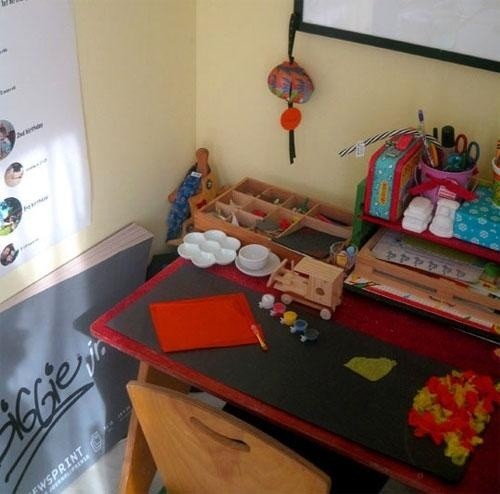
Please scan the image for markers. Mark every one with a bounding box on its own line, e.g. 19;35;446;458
441;125;455;166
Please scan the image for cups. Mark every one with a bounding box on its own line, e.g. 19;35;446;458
492;157;500;209
330;242;358;270
238;243;270;270
420;154;476;208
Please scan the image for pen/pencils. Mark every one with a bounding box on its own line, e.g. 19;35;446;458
238;295;267;350
417;111;438;170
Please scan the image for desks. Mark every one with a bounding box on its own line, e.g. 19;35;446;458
87;256;500;494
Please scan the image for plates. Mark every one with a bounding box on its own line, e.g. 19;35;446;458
235;252;281;278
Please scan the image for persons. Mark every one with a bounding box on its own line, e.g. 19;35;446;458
0;126;12;155
1;247;15;265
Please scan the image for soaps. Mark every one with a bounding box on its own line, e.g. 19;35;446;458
433;216;451;232
409;196;431;213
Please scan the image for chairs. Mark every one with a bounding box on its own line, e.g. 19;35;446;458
123;378;332;494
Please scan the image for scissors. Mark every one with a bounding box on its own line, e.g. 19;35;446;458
455;134;480;170
